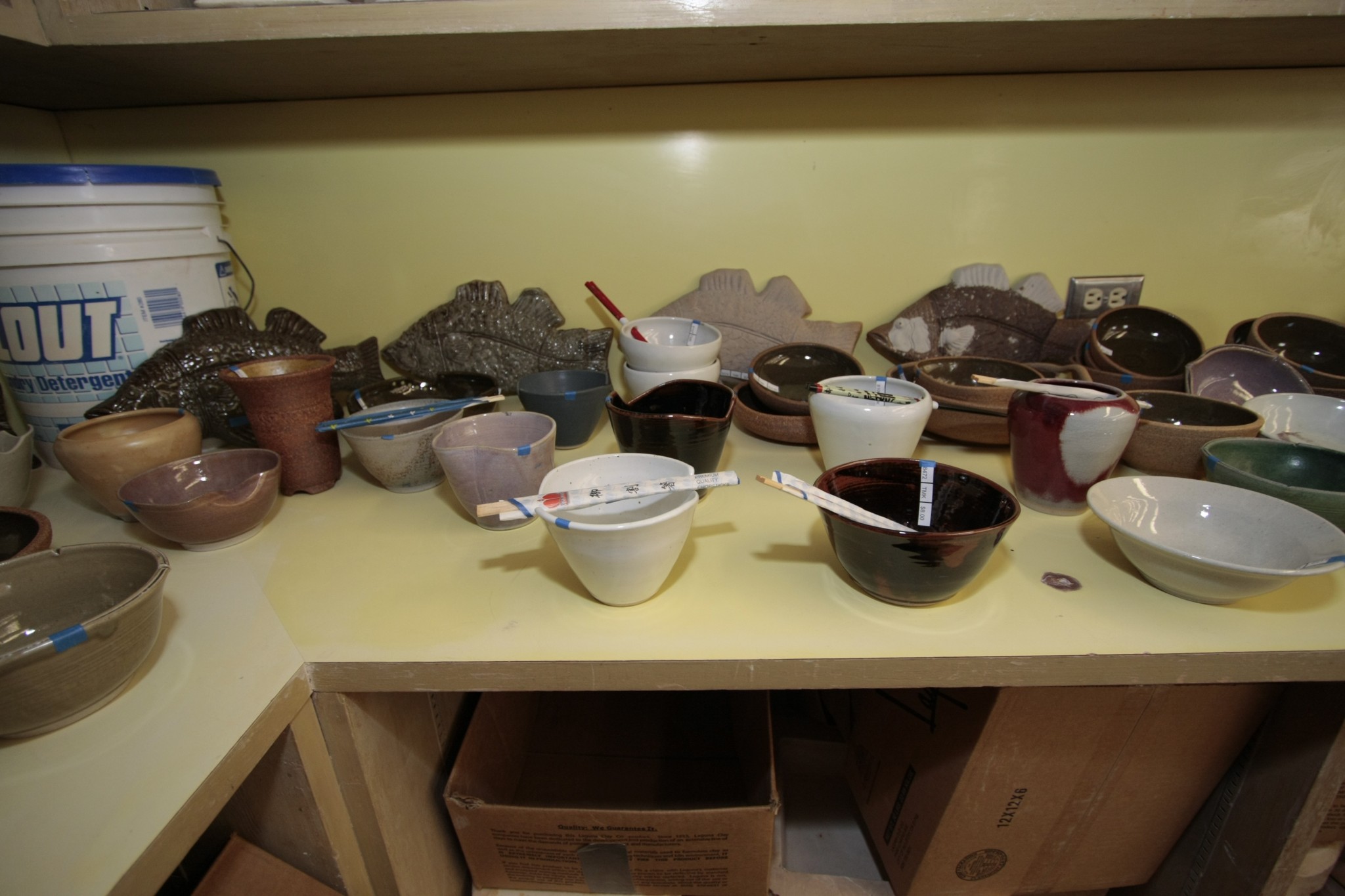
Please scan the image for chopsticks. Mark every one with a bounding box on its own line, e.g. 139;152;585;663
756;475;914;532
317;393;506;431
804;381;1008;418
585;281;649;343
477;471;739;518
970;372;1154;411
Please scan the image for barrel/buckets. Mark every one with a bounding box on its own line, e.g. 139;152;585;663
0;164;257;471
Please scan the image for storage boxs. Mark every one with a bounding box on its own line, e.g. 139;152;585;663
440;693;784;896
168;831;344;896
1285;778;1345;896
842;681;1278;896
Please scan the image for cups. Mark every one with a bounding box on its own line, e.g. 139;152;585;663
538;452;699;608
807;374;932;470
431;410;557;532
1008;377;1141;516
219;353;344;498
603;379;736;500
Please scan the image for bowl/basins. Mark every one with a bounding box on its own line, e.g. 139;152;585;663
0;408;280;740
338;316;736;606
732;305;1345;609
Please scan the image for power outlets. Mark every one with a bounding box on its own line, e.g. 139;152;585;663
1063;274;1146;318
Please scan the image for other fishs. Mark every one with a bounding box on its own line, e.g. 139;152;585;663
380;279;613;396
85;305;386;434
867;262;1091;366
648;268;863;382
